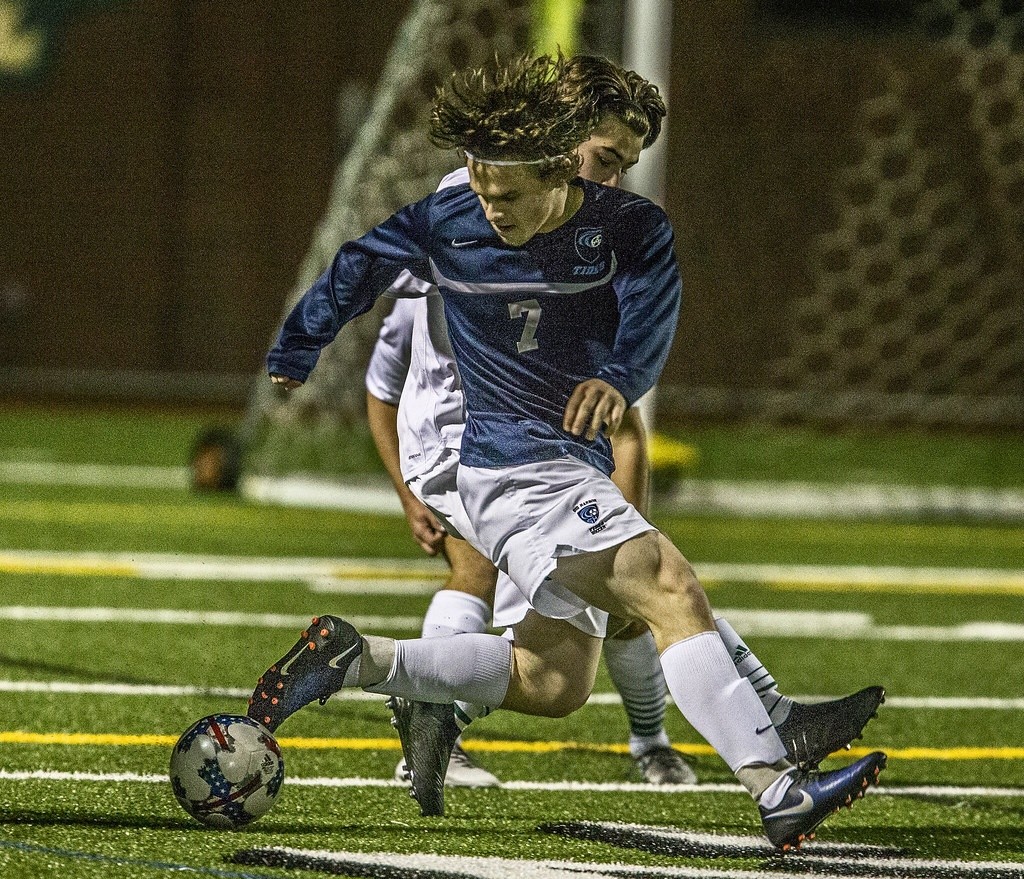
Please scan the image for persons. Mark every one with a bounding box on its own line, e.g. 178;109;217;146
268;43;888;851
385;53;885;817
366;298;697;788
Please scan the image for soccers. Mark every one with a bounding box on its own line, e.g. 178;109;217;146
169;712;285;829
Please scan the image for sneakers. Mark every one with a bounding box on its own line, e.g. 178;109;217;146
757;752;888;853
395;741;499;788
246;614;364;733
778;685;885;769
385;694;461;817
631;738;699;784
